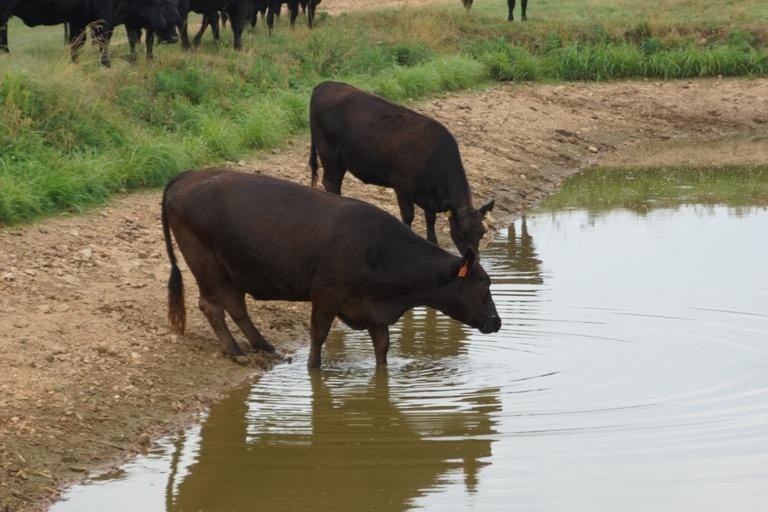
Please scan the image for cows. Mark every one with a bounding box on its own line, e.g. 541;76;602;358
309;81;495;261
462;0;531;22
0;0;322;65
161;166;502;370
